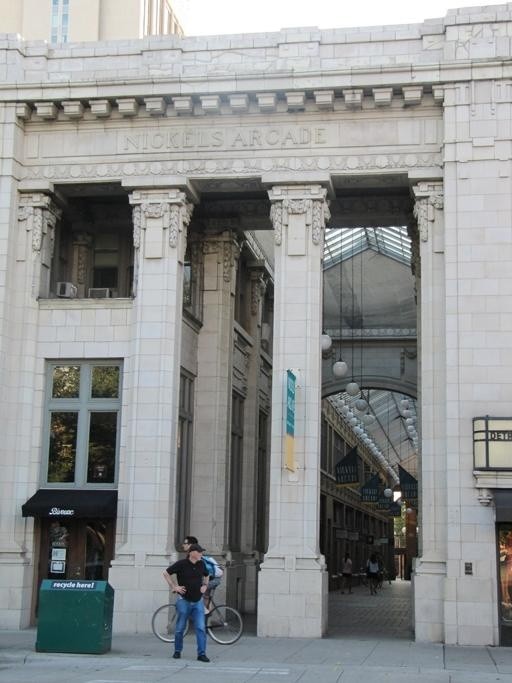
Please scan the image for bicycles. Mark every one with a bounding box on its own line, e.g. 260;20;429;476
152;586;244;645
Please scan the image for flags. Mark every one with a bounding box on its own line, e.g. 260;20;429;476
376;486;402;518
334;447;360;490
398;461;419;511
360;473;380;504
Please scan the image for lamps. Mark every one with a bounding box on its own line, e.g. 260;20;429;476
322;228;419;514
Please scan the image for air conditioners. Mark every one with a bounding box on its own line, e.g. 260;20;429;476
88;288;112;298
56;282;77;298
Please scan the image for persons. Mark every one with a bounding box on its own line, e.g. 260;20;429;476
367;551;385;595
341;552;354;594
182;536;223;615
162;544;209;662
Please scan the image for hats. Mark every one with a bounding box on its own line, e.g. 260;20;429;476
189;545;205;552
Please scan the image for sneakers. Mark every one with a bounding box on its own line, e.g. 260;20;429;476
173;652;180;658
198;655;209;662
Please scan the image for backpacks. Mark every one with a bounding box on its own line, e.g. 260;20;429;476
201;556;223;577
